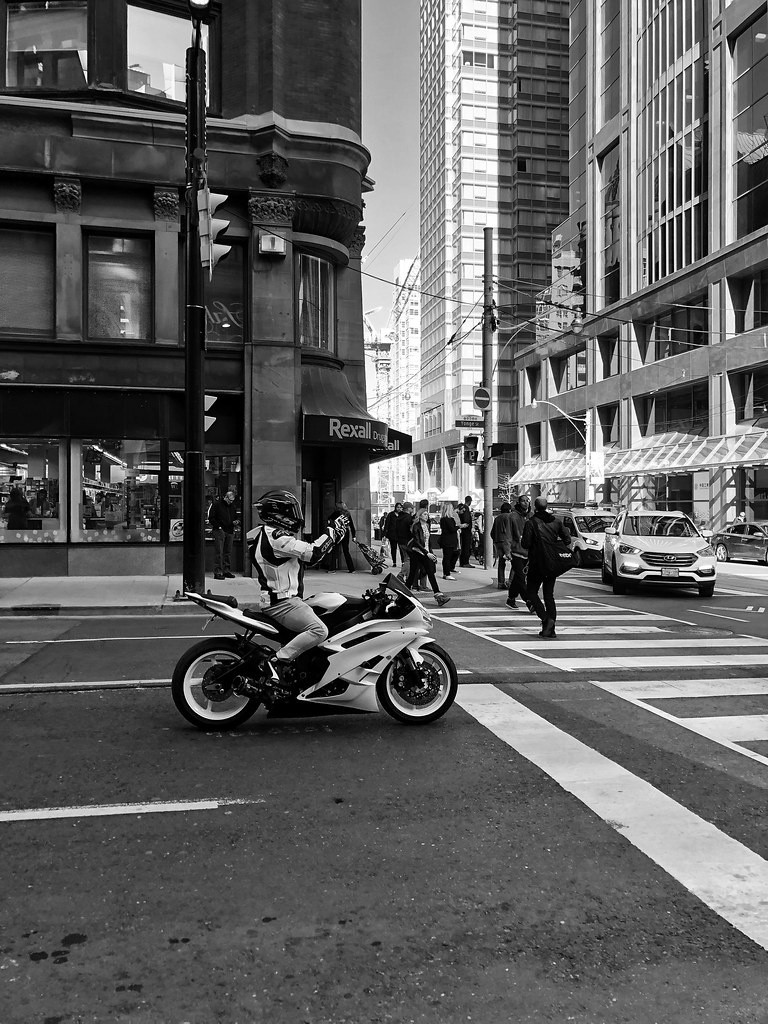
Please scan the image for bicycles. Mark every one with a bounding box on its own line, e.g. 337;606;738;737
473;540;496;568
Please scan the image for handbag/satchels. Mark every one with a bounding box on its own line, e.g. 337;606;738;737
541;540;575;576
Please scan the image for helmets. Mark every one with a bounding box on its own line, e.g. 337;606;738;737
254;490;305;533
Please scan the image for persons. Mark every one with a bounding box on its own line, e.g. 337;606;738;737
733;512;746;524
489;495;571;640
326;501;357;575
82;492;118;521
4;488;50;530
379;496;496;608
244;489;350;694
207;491;239;580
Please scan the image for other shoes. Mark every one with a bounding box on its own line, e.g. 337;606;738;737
543;617;553;636
506;599;518;610
460;562;474;568
450;569;459;572
347;570;355;573
499;583;505;588
214;574;224;579
412;583;419;589
397;573;405;582
539;630;556;638
326;570;334;573
392;564;396;567
443;575;454;579
420;586;433;592
225;572;235;577
506;580;512;586
529;605;533;612
436;594;450;607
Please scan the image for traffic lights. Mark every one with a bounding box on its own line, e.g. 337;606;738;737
463;435;479;464
204;392;217;433
197;185;233;282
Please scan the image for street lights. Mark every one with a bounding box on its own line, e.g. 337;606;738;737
529;398;591;504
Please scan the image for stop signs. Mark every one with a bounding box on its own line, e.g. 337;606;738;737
473;388;491;410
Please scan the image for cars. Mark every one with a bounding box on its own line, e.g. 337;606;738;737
706;521;768;566
430;512;443;536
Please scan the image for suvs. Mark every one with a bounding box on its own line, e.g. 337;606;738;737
602;511;719;599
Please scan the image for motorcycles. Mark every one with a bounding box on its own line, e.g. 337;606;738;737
168;537;459;731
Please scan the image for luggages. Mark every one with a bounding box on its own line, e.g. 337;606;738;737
352;539;389;575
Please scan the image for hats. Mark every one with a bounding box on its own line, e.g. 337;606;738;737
402;502;414;508
414;508;426;516
226;492;234;501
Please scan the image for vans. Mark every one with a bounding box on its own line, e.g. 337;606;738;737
547;500;618;567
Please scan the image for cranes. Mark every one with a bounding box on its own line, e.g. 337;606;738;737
364;252;420;516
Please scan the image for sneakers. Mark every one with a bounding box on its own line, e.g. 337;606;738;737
267;655;291;687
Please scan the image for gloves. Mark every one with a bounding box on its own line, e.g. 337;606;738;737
326;511;350;545
